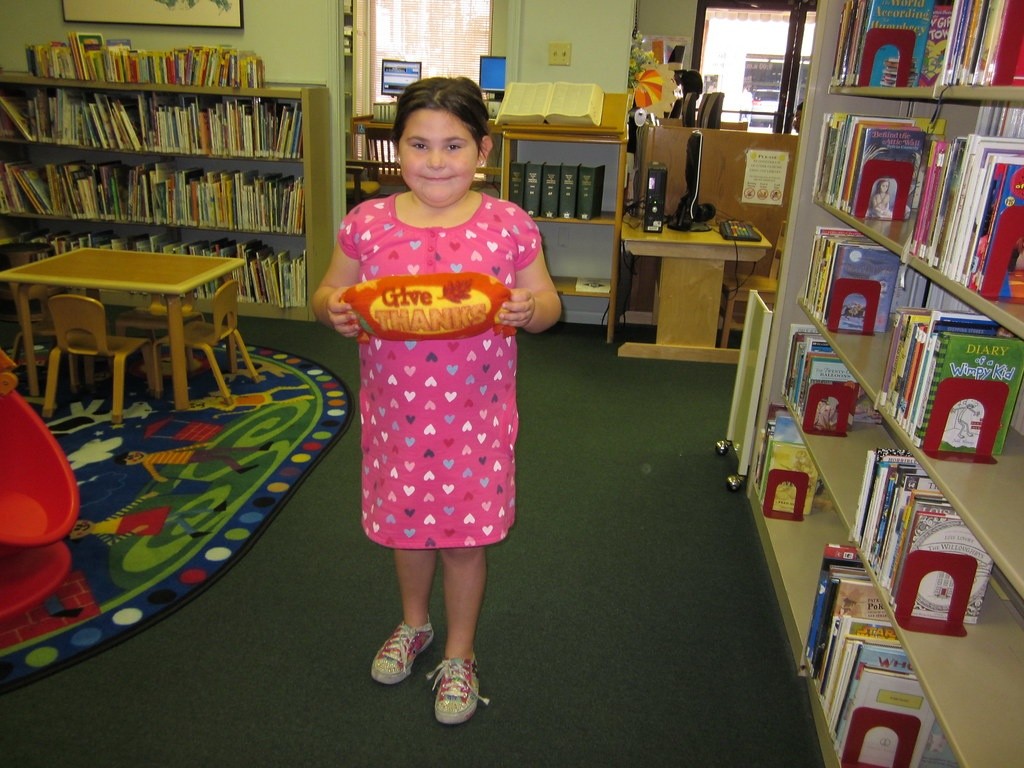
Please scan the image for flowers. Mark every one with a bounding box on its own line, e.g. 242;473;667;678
627;29;660;89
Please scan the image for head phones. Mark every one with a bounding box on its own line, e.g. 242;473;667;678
692;204;716;223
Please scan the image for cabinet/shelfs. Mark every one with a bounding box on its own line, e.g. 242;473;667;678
340;0;369;160
746;0;1024;768
500;93;631;344
0;71;335;322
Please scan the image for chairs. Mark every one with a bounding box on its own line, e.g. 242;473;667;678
364;127;412;196
344;156;400;210
718;221;787;349
1;242;262;424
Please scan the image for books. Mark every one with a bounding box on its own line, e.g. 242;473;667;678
880;268;1024;456
575;277;611;293
0;229;308;309
25;31;265;88
509;158;607;219
754;400;818;515
853;445;994;624
494;79;604;128
806;544;961;768
909;100;1024;305
353;103;401;176
831;0;1024;86
0;157;306;236
802;225;901;332
0;87;303;159
781;323;883;431
812;108;946;222
473;158;487;182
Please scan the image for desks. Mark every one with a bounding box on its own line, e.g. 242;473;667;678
617;210;772;362
0;248;246;411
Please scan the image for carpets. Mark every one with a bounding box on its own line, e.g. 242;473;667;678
0;337;354;696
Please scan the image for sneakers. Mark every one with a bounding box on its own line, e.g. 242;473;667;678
371;614;434;683
427;653;490;724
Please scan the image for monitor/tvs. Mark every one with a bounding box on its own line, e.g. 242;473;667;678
381;59;422;104
667;130;712;232
480;56;506;101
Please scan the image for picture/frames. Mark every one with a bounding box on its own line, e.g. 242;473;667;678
61;0;245;30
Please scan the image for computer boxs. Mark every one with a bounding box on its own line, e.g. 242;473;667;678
643;160;668;233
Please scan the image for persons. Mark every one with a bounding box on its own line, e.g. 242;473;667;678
793;101;803;132
310;75;562;725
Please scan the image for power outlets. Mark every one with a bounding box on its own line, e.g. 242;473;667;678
548;41;571;66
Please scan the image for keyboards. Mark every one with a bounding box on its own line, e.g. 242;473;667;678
718;221;762;242
